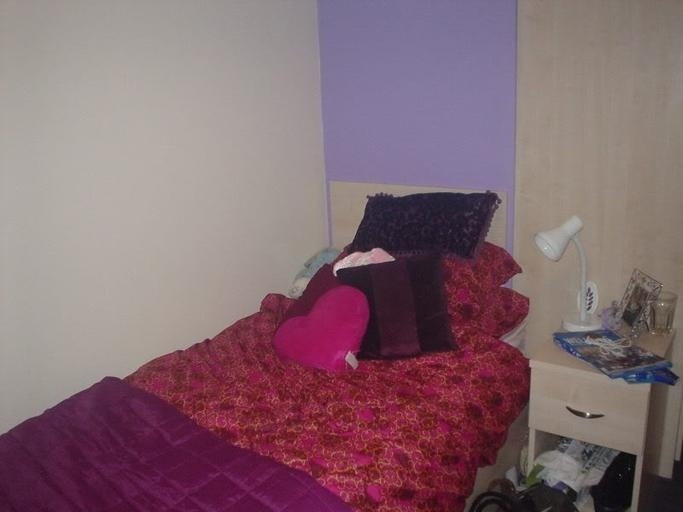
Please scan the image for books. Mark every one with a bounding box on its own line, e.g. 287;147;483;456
554;329;679;386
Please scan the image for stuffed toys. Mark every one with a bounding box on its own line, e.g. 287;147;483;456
288;245;342;301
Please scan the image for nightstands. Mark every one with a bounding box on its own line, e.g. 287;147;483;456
527;328;677;512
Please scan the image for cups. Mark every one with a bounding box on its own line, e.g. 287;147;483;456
648;291;679;337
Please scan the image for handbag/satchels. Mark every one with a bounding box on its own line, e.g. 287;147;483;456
553;326;681;385
470;483;579;512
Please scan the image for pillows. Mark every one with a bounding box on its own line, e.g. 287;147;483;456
334;253;463;362
447;235;530;347
270;283;372;373
346;190;503;262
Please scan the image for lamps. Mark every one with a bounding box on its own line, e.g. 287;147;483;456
534;214;602;333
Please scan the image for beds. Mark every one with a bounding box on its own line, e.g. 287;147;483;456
1;322;530;512
